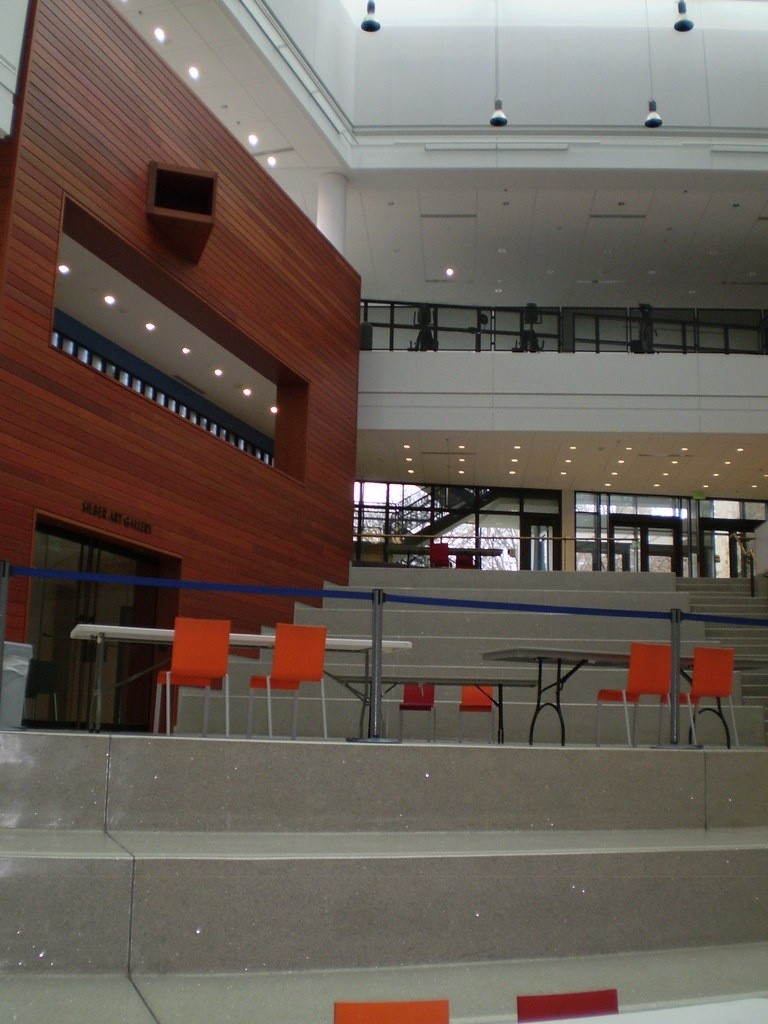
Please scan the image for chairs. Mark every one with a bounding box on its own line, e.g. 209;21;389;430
398;684;436;743
454;552;479;569
153;618;232;738
656;647;739;747
458;686;497;744
595;643;672;749
430;543;452;570
333;990;619;1024
247;623;328;740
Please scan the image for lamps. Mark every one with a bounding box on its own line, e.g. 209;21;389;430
360;0;695;128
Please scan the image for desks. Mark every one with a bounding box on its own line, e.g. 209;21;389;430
333;675;539;744
70;624;414;739
483;647;731;750
